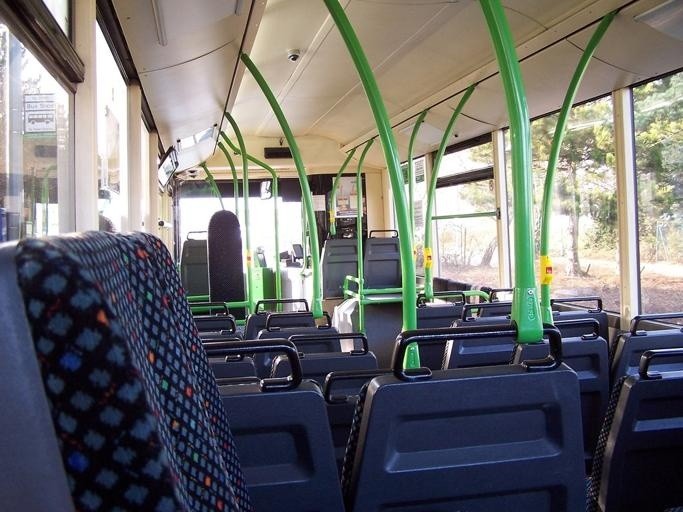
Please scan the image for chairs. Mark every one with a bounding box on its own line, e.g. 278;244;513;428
1;223;683;510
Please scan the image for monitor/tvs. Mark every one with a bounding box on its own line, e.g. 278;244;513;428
292;244;304;257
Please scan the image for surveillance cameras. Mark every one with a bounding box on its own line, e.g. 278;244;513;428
288;49;300;62
